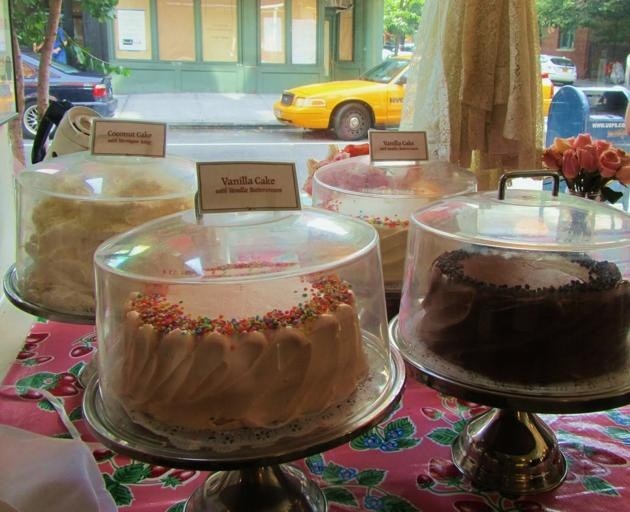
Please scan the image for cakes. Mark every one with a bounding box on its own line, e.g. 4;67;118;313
417;242;630;385
327;187;448;286
106;261;369;431
18;166;194;312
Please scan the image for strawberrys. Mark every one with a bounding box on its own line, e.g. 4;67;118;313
306;400;630;509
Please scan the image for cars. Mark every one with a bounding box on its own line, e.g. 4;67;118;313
1;46;119;140
540;54;578;85
271;53;556;142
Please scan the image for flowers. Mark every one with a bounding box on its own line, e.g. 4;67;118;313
541;132;630;205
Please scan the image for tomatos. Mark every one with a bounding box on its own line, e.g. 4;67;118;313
17;334;195;482
